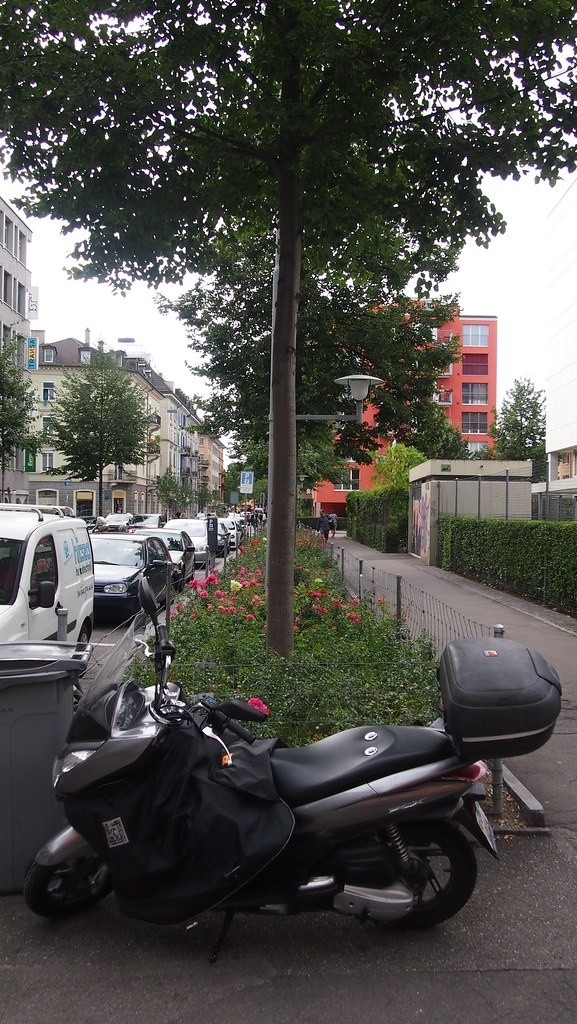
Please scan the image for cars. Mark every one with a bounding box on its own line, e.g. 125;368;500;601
0;491;265;649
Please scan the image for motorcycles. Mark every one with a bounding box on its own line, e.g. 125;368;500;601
23;579;564;969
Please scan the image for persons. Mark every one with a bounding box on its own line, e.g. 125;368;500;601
317;509;332;543
328;510;338;539
70;499;268;547
